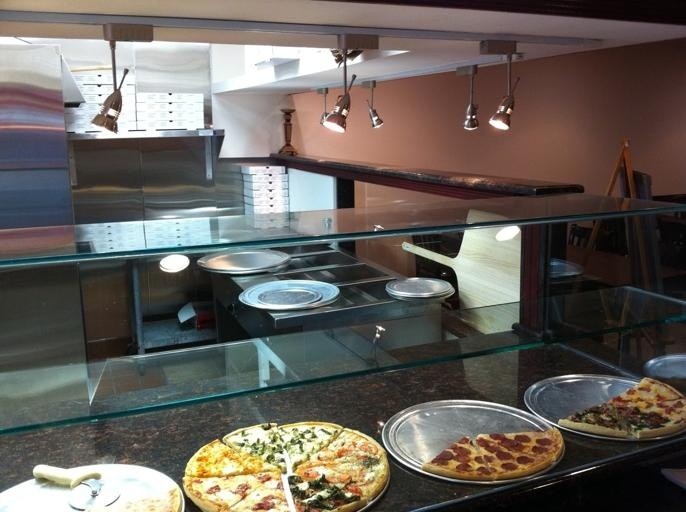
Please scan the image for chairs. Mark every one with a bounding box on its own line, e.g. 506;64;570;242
568;223;601;252
414;235;444;278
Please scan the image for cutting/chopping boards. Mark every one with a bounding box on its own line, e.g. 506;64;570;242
401;208;522;336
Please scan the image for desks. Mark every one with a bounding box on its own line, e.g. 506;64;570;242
565;243;681;338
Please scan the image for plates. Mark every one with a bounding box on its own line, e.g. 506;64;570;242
0;463;186;512
238;279;340;311
196;248;291;275
642;353;686;380
549;257;586;279
523;373;686;442
381;398;566;484
385;277;456;299
356;468;390;512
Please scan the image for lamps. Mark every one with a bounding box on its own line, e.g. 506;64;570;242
324;35;379;133
92;24;153;133
359;81;384;129
455;65;480;131
481;39;521;131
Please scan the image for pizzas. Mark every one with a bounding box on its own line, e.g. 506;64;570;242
181;420;390;512
556;377;686;440
83;485;184;512
422;425;565;482
